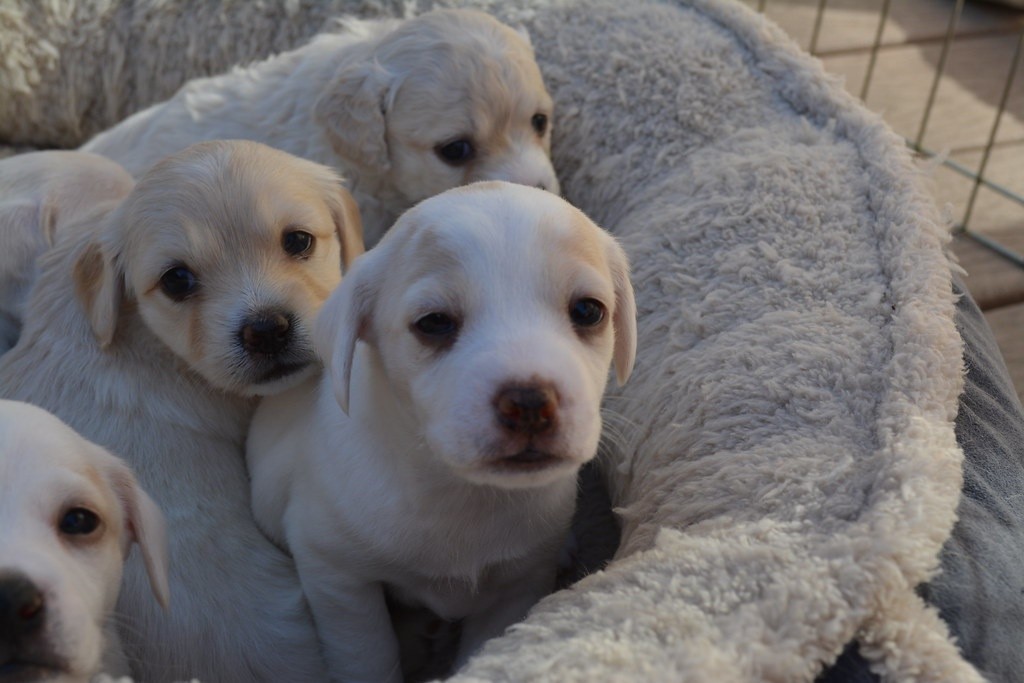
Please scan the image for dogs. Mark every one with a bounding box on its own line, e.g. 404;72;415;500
244;179;638;683
0;138;366;683
0;149;136;359
77;8;561;253
0;399;172;683
